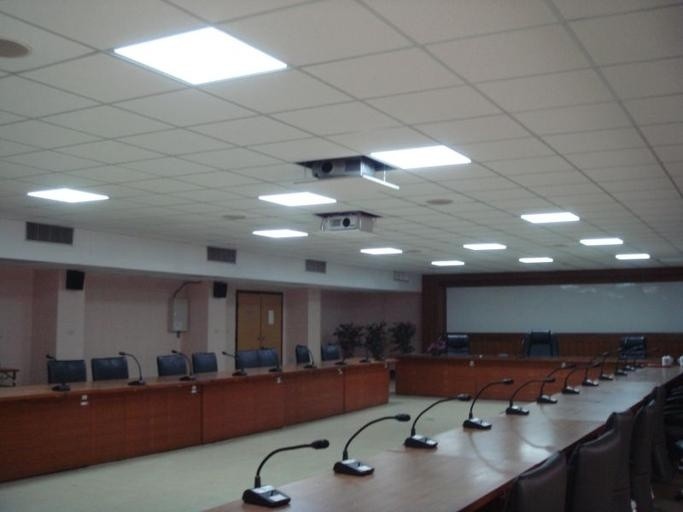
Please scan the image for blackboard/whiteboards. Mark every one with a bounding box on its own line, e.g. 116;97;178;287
445;282;683;335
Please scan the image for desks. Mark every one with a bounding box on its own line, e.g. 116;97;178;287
216;361;683;511
392;348;628;404
2;354;394;487
519;330;561;356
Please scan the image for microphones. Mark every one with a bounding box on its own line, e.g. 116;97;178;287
403;393;471;449
463;378;514;430
171;349;197;381
333;414;410;475
222;352;247;376
243;439;330;508
537;343;660;402
118;351;147;386
258;337;371;372
505;378;556;415
46;354;74;391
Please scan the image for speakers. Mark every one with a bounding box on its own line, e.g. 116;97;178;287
66;270;85;289
213;280;227;297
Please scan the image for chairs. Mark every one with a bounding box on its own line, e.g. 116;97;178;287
44;358;88;382
619;335;648;358
573;427;632;512
92;356;130;380
293;344;312;364
513;451;570;511
632;398;660;512
445;333;472;353
320;343;341;361
154;353;189;376
605;407;635;443
191;350;219;372
233;349;259;368
258;347;278;367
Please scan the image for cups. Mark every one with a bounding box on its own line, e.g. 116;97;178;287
678;356;682;366
660;356;672;366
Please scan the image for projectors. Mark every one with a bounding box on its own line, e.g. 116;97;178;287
319;214;377;240
311;156;375;177
